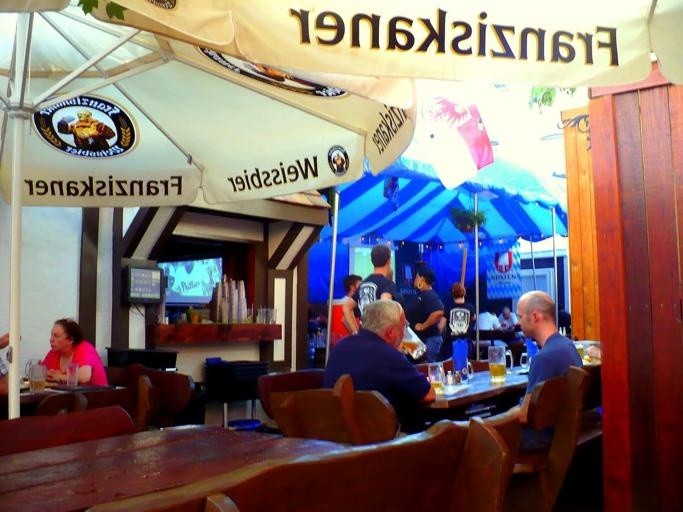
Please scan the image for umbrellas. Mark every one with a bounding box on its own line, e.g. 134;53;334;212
0;0;416;419
115;0;683;88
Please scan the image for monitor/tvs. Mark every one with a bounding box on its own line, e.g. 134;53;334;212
121;265;164;306
155;255;225;308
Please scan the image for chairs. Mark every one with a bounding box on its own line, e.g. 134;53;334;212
0;322;607;512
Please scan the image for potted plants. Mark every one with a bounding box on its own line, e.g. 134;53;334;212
448;207;486;232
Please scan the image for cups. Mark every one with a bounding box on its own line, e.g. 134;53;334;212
487;346;514;383
451;334;474;382
428;363;442;389
65;363;78;387
402;327;425;360
22;358;47;392
213;278;248;324
256;308;280;326
519;336;539;371
177;311;187;321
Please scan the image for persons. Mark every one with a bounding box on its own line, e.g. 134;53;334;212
511;290;583;450
0;332;22;395
38;319;108;387
331;246;570;362
322;299;435;434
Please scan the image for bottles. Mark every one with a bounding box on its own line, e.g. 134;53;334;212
448;371;462;385
